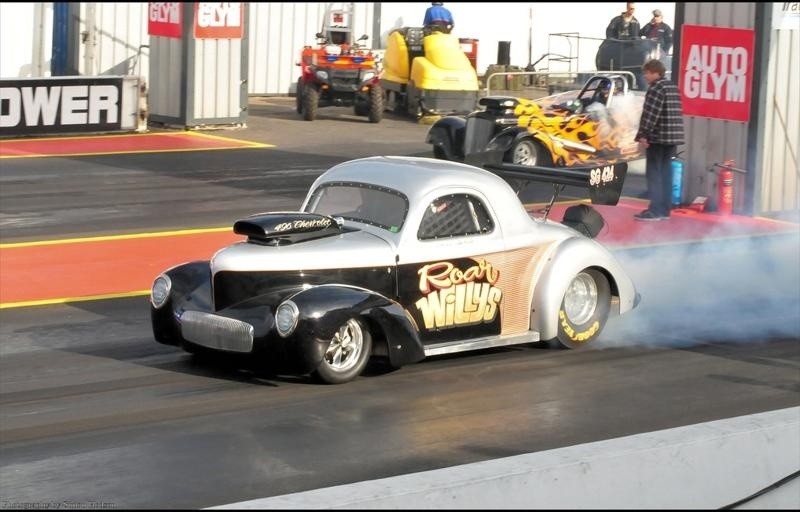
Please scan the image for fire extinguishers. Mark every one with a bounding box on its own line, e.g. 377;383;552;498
670;151;686;205
716;158;735;215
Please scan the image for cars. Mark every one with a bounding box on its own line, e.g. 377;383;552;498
148;153;641;385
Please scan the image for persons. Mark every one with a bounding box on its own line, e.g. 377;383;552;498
638;8;673;56
606;2;641;39
422;3;454;26
595;81;620;105
632;58;682;221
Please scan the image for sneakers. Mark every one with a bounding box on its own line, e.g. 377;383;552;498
634;209;671;221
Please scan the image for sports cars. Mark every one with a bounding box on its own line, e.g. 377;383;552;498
425;70;646;168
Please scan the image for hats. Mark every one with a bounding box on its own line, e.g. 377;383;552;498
653;9;662;16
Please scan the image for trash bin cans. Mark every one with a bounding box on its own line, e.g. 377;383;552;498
486;64;523;91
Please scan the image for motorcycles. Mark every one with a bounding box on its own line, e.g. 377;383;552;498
295;11;383;124
383;27;479;118
